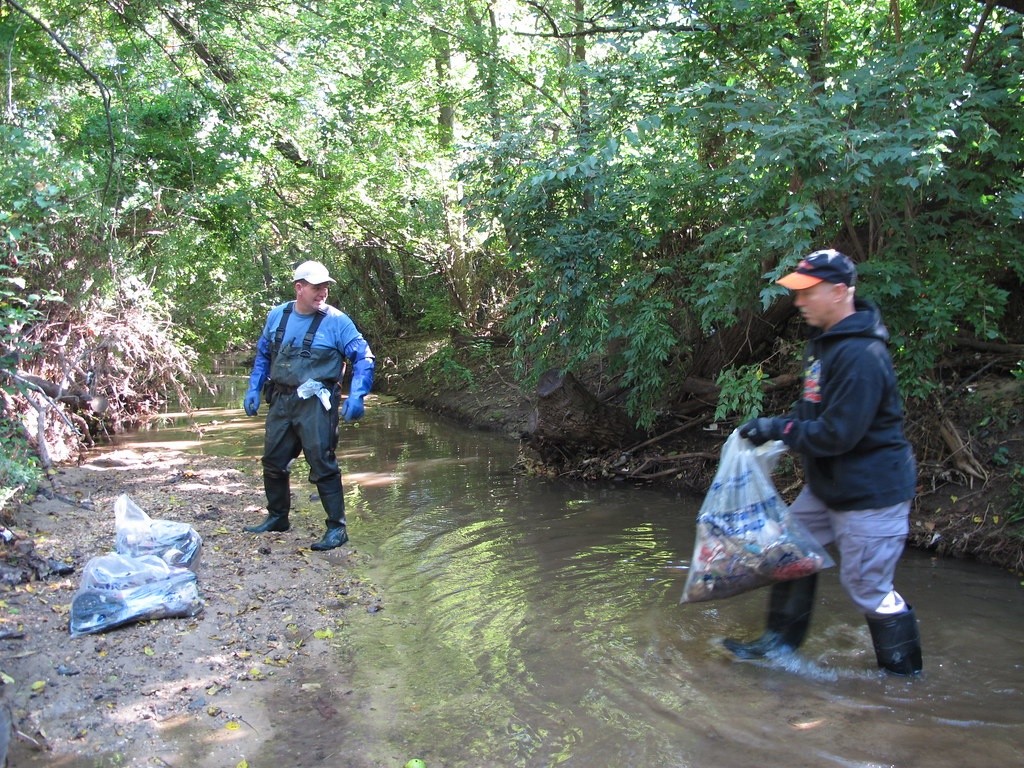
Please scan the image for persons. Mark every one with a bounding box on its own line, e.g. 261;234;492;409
243;260;375;551
722;248;923;677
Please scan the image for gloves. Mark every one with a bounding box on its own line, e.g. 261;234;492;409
243;335;272;416
740;416;790;446
341;341;376;424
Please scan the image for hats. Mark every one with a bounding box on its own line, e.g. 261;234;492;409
292;260;337;285
775;249;858;290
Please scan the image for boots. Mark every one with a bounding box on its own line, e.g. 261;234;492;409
708;572;821;662
866;605;924;677
242;467;291;534
310;473;349;551
